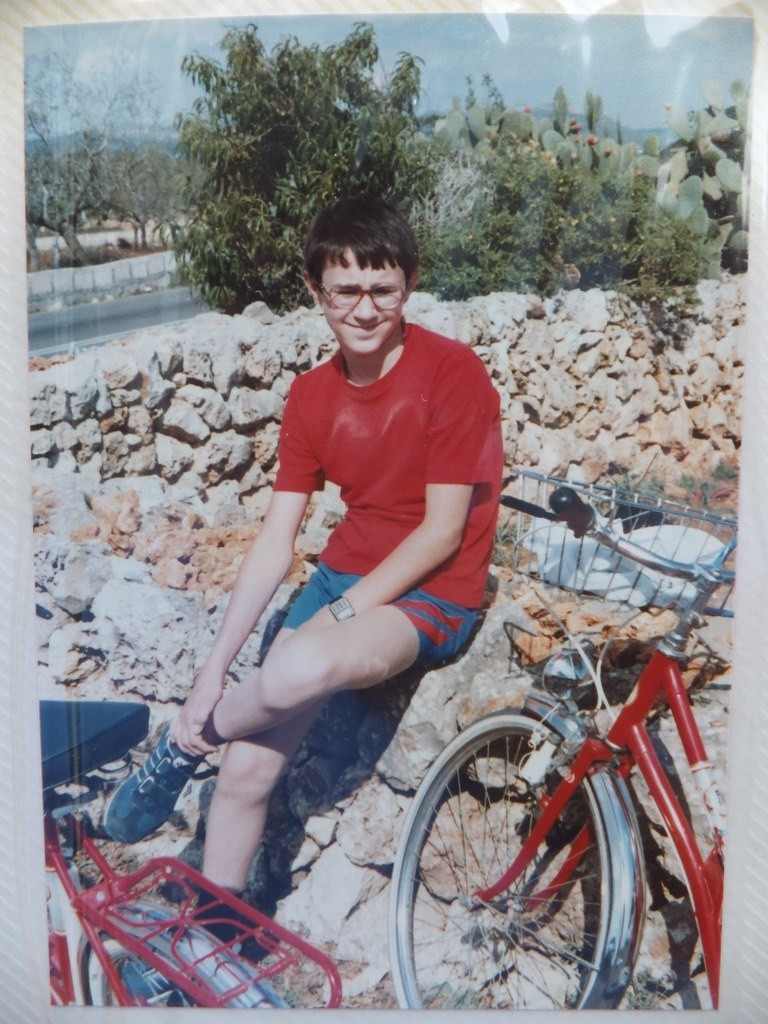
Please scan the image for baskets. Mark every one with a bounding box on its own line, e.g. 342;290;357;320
511;467;738;616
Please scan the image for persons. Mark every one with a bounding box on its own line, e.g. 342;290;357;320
102;193;503;999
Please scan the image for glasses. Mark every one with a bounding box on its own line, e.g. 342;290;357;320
311;277;409;311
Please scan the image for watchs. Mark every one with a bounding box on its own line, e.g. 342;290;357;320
327;594;355;627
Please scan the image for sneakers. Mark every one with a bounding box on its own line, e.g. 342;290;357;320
113;913;225;995
102;722;208;845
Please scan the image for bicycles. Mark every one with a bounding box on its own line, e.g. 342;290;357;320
40;700;343;1012
388;467;737;1010
